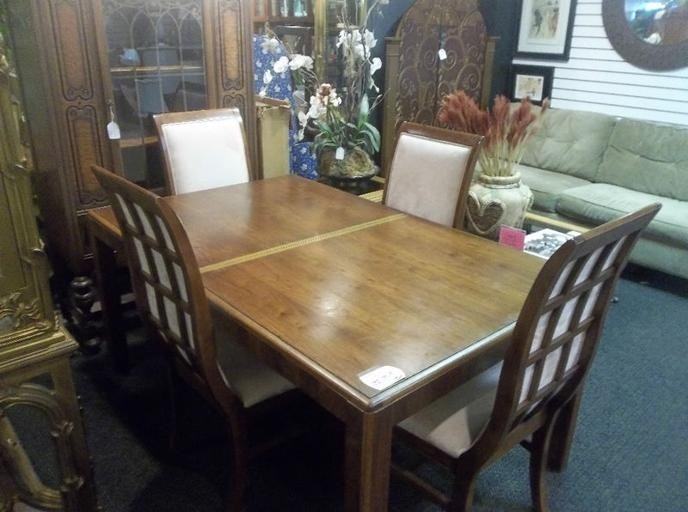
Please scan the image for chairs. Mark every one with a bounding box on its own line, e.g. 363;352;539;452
369;199;664;512
149;100;252;202
89;162;343;510
377;116;486;232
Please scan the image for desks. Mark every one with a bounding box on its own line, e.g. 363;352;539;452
352;185;592;259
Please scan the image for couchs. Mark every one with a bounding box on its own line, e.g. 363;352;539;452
472;95;687;294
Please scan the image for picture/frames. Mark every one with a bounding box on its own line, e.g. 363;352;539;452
501;63;556;106
507;0;580;63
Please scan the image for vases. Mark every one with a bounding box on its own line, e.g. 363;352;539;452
463;173;537;246
313;161;379;193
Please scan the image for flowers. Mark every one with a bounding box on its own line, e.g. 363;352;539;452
253;0;394;152
